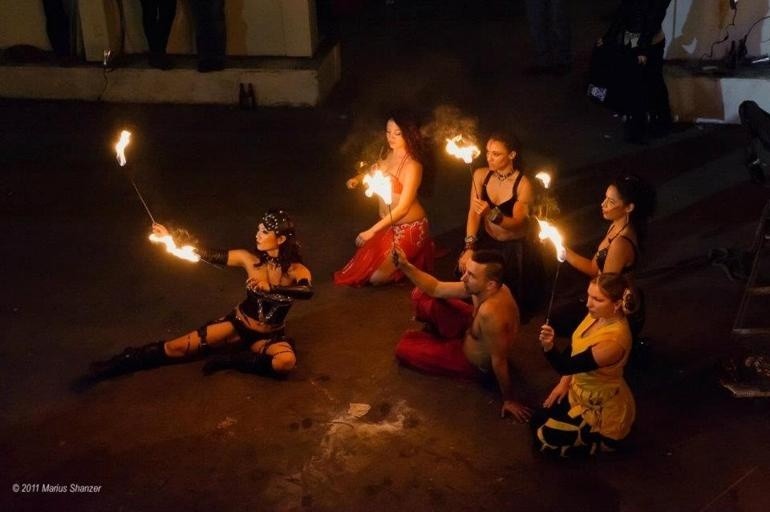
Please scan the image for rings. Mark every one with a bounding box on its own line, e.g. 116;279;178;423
477;209;480;212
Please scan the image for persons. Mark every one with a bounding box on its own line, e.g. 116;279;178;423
455;133;549;318
595;0;670;147
332;111;434;287
543;176;657;379
66;209;312;393
533;271;638;468
390;238;537;425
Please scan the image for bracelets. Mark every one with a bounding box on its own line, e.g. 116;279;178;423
463;236;479;250
488;206;503;224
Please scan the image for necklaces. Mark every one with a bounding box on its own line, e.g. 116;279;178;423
493;168;516;180
263;254;281;267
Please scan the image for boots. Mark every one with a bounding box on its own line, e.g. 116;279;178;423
203;352;282;378
82;339;173;384
